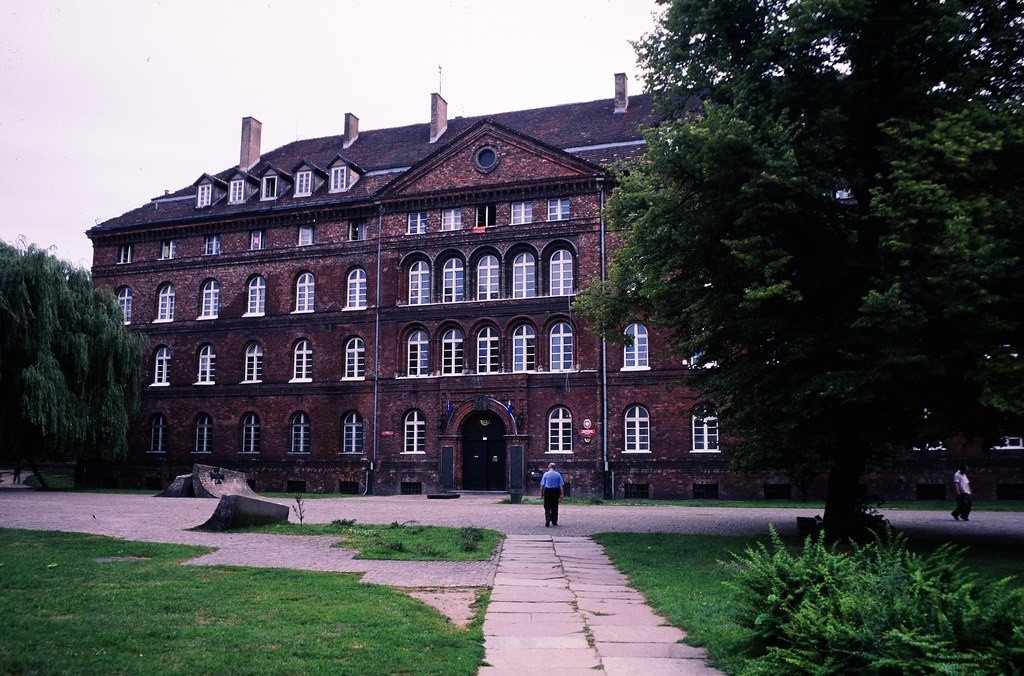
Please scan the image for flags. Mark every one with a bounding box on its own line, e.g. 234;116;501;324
507;400;512;416
446;396;451;415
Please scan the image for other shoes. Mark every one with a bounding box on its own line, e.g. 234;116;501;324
951;511;959;521
552;522;558;526
960;514;969;521
546;516;551;527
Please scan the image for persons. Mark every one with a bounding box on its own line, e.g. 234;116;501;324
951;465;971;520
13;466;21;484
540;463;565;527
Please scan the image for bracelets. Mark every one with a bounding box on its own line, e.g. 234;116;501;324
561;490;564;494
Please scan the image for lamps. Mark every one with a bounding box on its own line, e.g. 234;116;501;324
516;412;524;427
437;419;446;429
